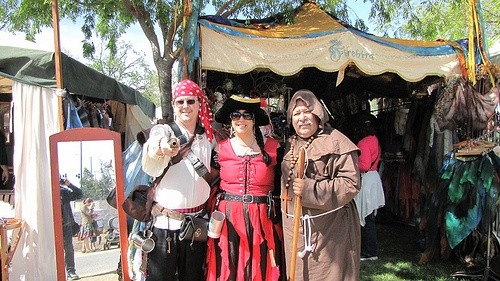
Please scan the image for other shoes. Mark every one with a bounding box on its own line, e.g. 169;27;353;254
360;252;378;262
66;269;79;280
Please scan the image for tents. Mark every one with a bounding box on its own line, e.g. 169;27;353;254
0;47;155;281
173;0;490;265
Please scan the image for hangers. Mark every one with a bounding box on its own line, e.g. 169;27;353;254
455;138;497;157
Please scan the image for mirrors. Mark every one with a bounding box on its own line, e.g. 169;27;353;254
48;128;130;281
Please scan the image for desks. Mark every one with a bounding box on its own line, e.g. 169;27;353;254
0;218;26;281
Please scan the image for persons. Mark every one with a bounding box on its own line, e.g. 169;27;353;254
137;79;385;281
58;174;100;280
0;129;8;184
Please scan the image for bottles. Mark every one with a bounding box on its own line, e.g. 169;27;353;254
494;115;500;145
488;122;494;141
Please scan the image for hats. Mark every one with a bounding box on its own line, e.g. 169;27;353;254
215;93;269;126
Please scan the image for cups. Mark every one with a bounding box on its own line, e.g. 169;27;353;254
130;232;154;253
208;210;225;238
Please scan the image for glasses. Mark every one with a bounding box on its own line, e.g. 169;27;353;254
174;99;199;105
230;111;255;120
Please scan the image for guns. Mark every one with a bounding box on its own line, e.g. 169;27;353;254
168;138;177;148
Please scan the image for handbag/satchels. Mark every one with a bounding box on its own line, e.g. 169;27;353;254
73;222;80;235
121;184;155;222
178;215;208;243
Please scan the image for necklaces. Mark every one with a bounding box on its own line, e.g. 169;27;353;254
286;134;315;189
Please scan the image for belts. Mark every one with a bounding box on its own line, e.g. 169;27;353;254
219;193;269;202
